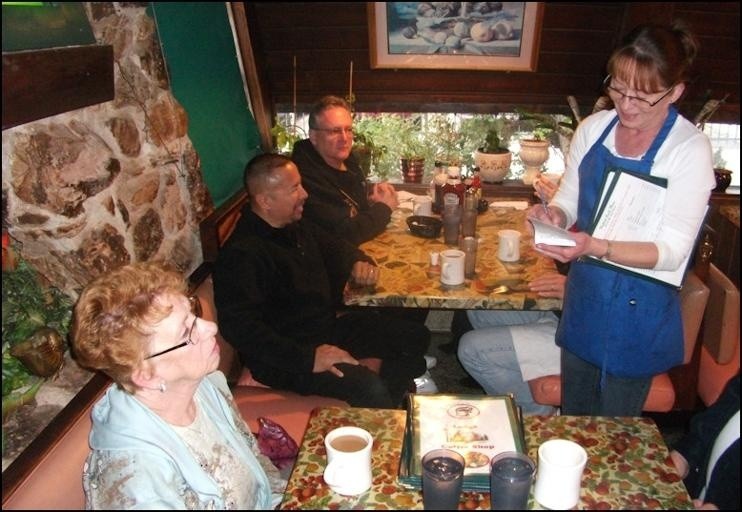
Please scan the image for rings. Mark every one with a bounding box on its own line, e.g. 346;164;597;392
369;270;375;274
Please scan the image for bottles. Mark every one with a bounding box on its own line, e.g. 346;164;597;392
430;251;437;267
431;160;482;212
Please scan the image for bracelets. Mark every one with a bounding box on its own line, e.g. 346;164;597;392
599;238;612;263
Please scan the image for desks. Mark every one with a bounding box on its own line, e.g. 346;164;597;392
719;205;740;228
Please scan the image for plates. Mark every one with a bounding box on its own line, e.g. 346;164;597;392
446;403;481;419
461;449;490;470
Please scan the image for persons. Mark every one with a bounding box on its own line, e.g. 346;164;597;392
288;95;430;324
70;261;288;511
212;152;430;409
459;173;578;416
521;16;717;416
671;368;740;510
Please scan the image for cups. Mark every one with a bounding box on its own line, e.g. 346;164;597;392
488;450;538;510
412;196;432;217
438;248;466;287
442;196;478;277
420;449;465;511
496;230;522;262
322;425;375;497
535;436;588;509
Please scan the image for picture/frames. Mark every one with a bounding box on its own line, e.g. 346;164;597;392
366;0;544;73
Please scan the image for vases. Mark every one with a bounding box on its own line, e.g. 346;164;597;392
396;146;425;183
710;162;734;194
517;137;549;186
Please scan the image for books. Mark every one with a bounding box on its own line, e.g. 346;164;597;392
395;390;528;493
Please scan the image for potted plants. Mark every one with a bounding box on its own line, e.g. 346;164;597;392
349;126;389;183
471;128;510;186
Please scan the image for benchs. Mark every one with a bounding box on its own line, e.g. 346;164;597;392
193;181;382;395
0;262;352;511
693;217;741;411
523;260;710;416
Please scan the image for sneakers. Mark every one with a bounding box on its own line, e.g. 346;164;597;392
413;353;439;395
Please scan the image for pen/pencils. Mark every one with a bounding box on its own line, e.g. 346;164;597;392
539;188;554;226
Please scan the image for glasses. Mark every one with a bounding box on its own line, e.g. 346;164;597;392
141;294;204;361
602;71;676;112
310;126;354;136
